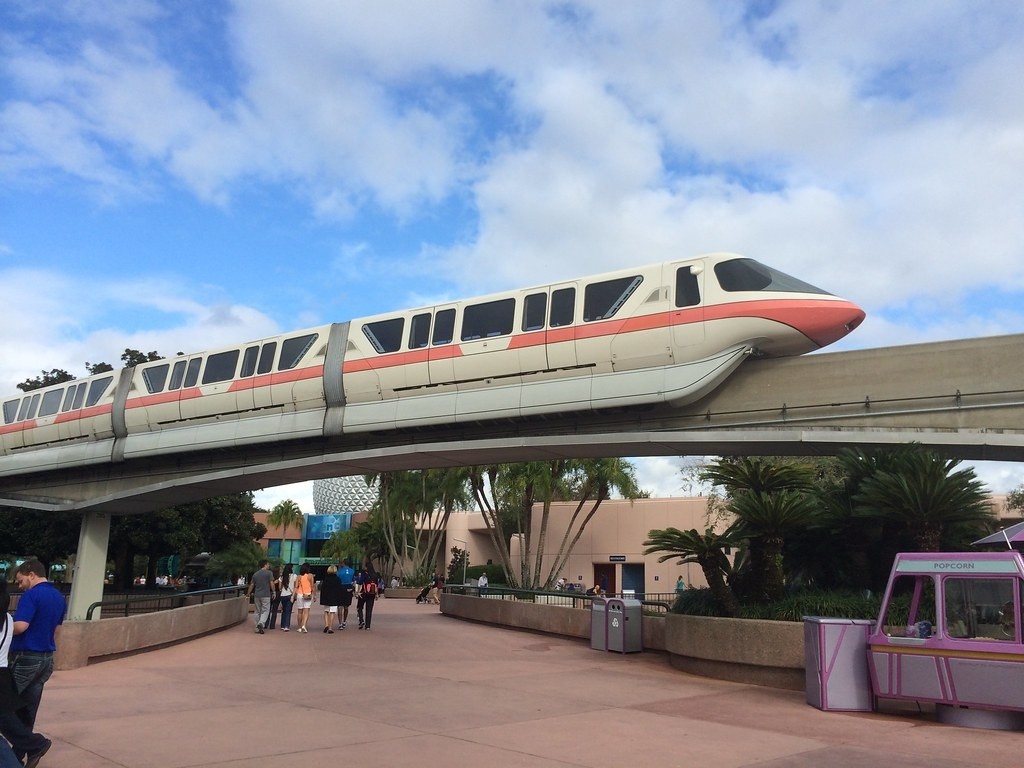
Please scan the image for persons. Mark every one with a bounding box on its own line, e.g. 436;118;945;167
676;575;687;593
553;578;567;591
354;562;378;631
290;562;316;633
132;575;245;593
586;584;600;596
244;559;276;634
352;572;488;607
278;563;292;632
318;565;343;633
334;559;355;630
0;576;52;768
6;558;66;767
263;566;283;629
600;571;608;598
568;583;575;591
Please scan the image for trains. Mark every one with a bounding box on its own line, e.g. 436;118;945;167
0;254;866;455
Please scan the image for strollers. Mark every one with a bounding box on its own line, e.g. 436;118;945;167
415;585;433;604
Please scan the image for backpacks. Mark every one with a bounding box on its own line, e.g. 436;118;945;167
362;572;378;597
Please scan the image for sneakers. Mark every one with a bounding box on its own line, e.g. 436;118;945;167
365;626;371;630
302;626;308;633
358;622;365;629
323;626;329;633
328;630;334;634
339;624;343;630
297;628;302;633
23;737;52;768
257;622;264;634
280;626;290;632
342;622;347;628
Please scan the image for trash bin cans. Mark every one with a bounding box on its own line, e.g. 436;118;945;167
564;584;573;589
607;599;643;655
574;584;584;589
591;598;620;650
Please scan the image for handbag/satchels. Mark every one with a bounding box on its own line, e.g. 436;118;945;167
0;667;20;719
302;593;313;601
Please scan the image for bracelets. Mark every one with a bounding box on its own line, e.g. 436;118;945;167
291;595;295;599
272;591;276;594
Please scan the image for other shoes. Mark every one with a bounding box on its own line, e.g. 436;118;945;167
270;624;275;629
